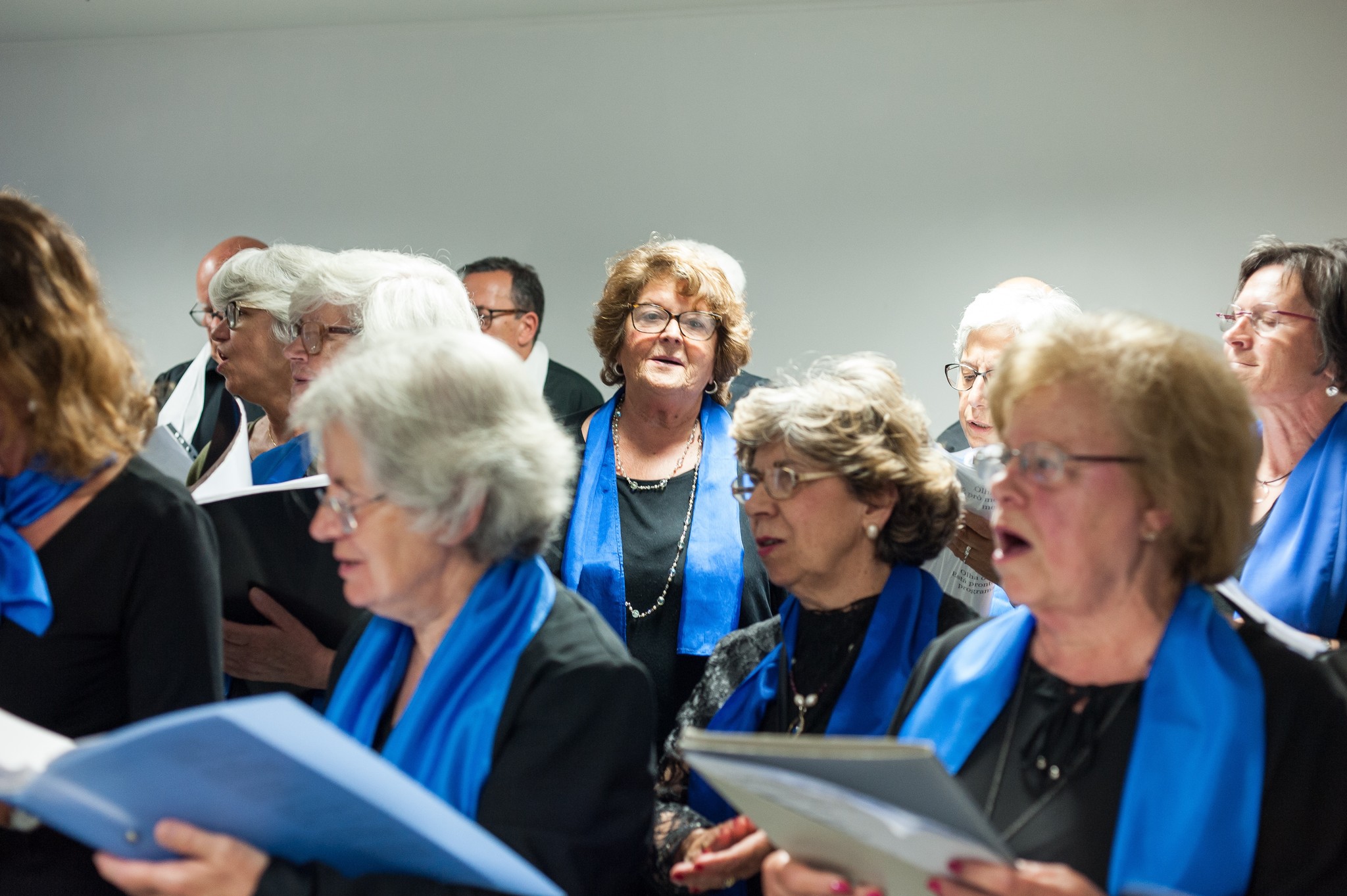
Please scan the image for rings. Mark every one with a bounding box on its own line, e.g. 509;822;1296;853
963;556;968;563
965;545;972;556
725;871;735;888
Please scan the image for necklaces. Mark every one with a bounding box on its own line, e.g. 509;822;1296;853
613;405;697;491
268;422;277;447
613;395;703;620
982;639;1143;841
786;643;854;739
1253;469;1291;503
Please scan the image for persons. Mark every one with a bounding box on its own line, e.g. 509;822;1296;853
458;256;604;450
92;324;660;896
185;243;306;487
933;277;1085;614
1224;234;1347;679
636;349;980;896
763;312;1347;895
542;244;786;795
149;236;268;456
671;238;775;412
219;246;481;718
0;189;226;896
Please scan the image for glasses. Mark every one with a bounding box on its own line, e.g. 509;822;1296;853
475;305;528;331
731;466;841;504
225;301;255;330
1216;303;1318;333
944;363;998;391
975;441;1142;492
189;302;224;328
626;302;722;341
289;321;361;356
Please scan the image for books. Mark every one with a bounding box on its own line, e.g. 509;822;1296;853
677;723;1012;862
